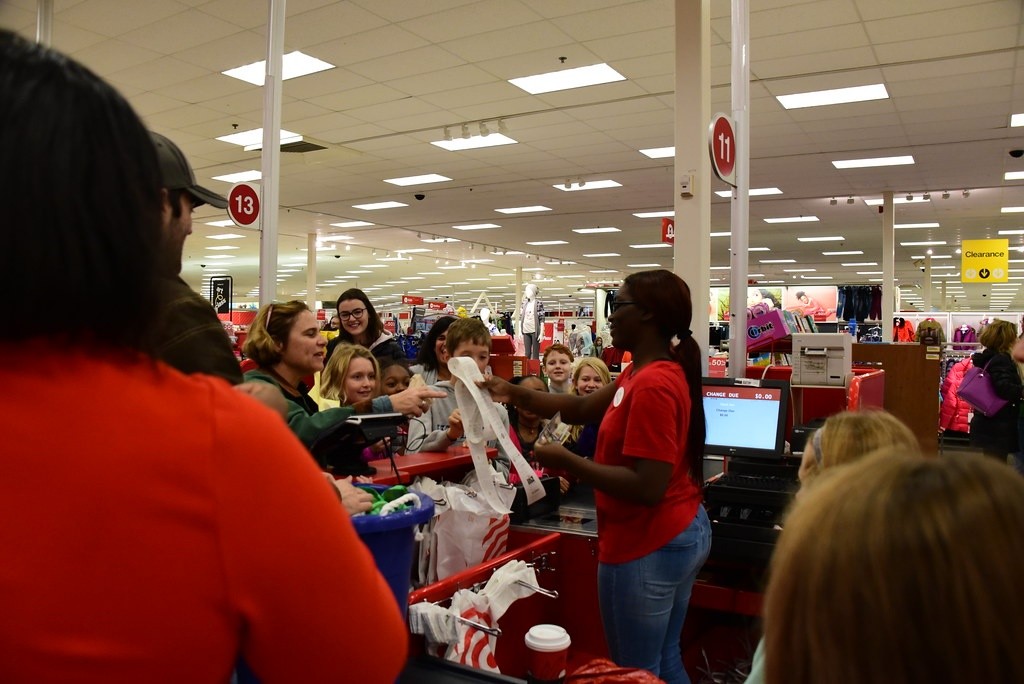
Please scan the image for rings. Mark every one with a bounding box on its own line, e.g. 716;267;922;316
421;400;428;408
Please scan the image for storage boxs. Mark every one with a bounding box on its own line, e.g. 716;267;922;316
746;308;819;351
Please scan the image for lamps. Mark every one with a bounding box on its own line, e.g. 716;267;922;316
847;195;855;204
372;232;563;265
941;191;950;200
578;177;585;187
565;177;572;188
963;189;972;198
830;197;838;205
478;121;490;137
906;192;914;201
460;123;471;139
444;127;453;141
923;191;931;201
497;119;512;133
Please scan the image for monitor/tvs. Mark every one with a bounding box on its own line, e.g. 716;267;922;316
700;376;790;461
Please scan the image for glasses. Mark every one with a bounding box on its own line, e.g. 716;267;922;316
610;299;635;313
339;307;367;321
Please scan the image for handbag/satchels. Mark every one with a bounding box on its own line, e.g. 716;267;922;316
955;358;1010;417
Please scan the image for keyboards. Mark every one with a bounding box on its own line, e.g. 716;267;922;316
709;474;801;496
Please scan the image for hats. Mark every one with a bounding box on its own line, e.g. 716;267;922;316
149;130;229;209
529;284;539;297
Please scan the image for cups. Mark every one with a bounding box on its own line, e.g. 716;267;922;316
524;623;572;684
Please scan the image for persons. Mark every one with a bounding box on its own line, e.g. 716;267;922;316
242;299;447;463
746;411;919;684
0;27;411;684
448;268;713;684
753;290;781;311
970;321;1024;473
481;309;500;335
321;288;513;483
784;290;836;317
588;336;603;360
765;451;1024;684
520;284;545;359
510;345;611;499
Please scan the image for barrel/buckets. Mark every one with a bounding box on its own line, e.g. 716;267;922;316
351;483;435;620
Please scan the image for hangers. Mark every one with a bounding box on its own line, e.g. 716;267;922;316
945;353;974;363
866;323;882;338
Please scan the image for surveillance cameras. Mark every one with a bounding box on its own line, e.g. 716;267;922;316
414;195;425;200
1009;150;1024;158
201;265;205;267
983;295;986;297
335;255;340;258
910;303;913;305
